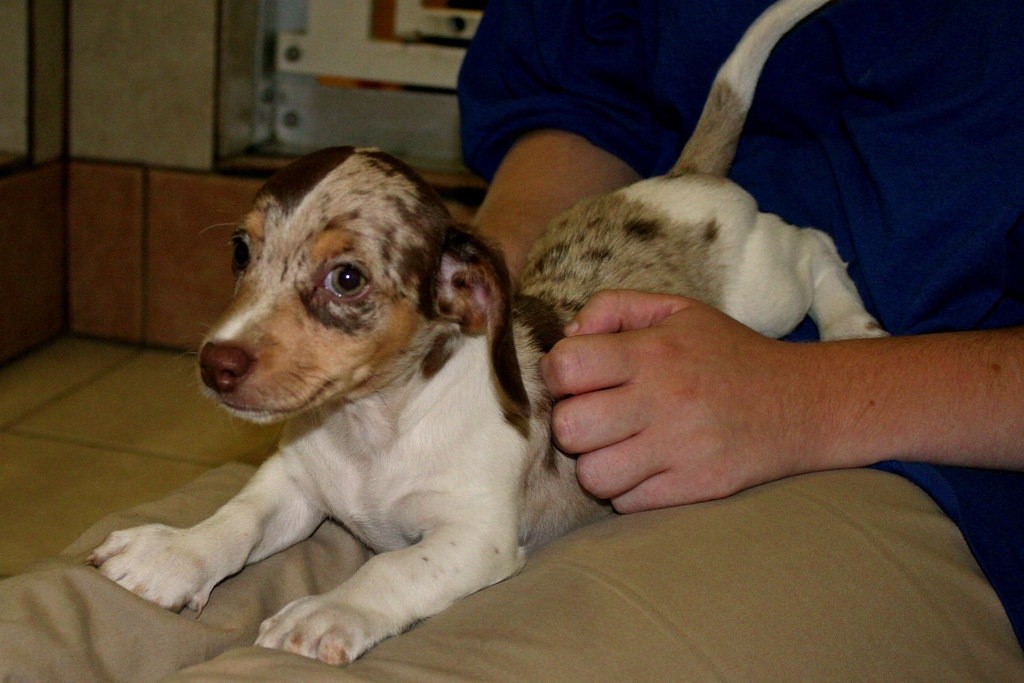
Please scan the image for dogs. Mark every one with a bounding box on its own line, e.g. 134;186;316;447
85;0;890;665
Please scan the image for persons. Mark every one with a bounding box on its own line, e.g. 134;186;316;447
0;0;1024;683
